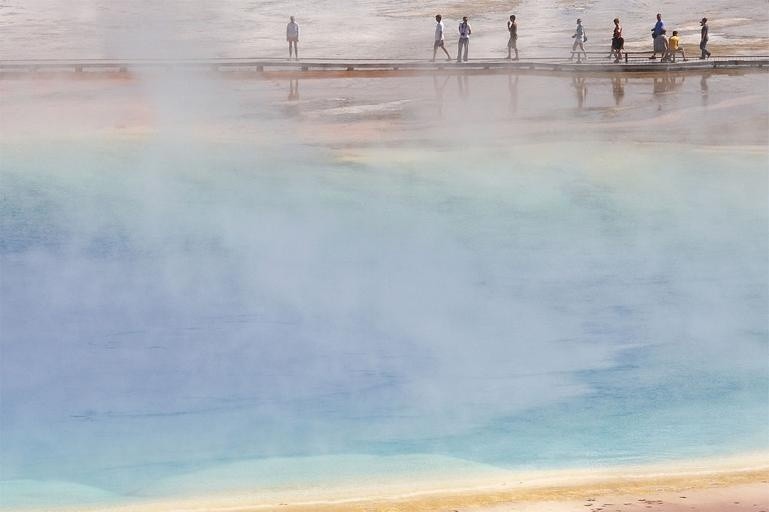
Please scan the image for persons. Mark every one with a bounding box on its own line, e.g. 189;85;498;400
506;15;520;61
432;74;450;116
612;70;686;109
571;18;588;61
649;14;664;59
609;19;623;65
429;15;450;61
288;79;299;101
652;30;669;63
507;74;519;114
697;17;712;60
611;33;625;65
666;31;689;63
457;74;470;97
573;76;587;107
285;16;300;60
699;72;712;104
455;17;472;63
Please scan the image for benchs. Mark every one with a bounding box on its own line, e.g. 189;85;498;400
569;49;681;64
